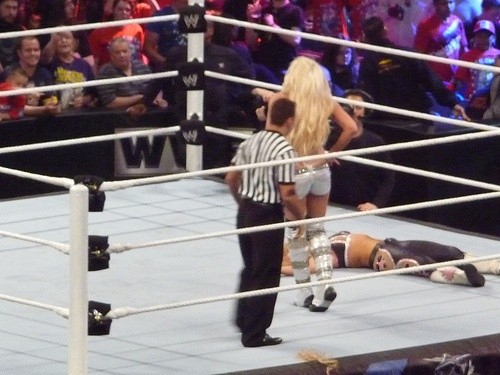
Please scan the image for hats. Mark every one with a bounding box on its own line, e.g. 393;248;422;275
472;20;496;35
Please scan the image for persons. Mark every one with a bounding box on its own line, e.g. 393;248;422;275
265;55;358;313
0;0;500;177
280;231;500;287
323;89;395;211
224;98;305;348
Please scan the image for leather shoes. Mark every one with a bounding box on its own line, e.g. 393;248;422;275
260;334;282;346
